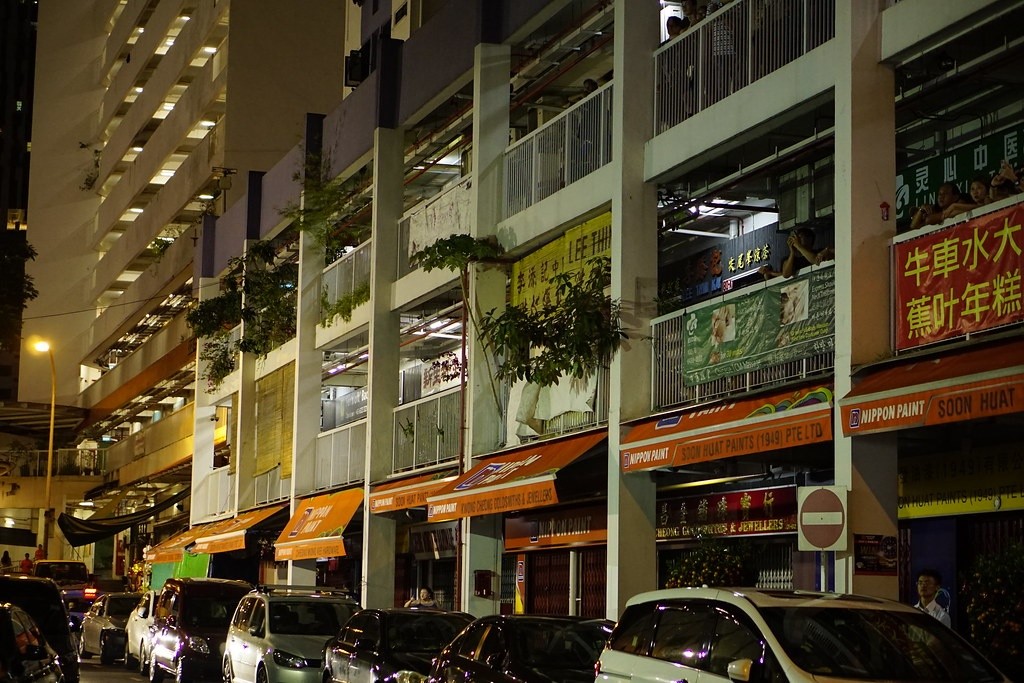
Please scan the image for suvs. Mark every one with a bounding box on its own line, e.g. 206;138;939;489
219;583;364;683
144;575;259;683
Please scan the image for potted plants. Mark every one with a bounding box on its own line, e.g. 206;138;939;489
20;442;101;477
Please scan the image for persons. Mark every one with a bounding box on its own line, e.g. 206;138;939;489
35;544;45;561
1;551;13;575
404;586;439;608
906;569;952;669
19;553;34;576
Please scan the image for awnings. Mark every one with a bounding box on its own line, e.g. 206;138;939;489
56;479;191;549
145;343;1024;562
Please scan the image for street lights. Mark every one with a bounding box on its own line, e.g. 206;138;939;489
31;338;58;558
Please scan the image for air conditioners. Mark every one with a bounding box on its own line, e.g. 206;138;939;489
527;107;566;151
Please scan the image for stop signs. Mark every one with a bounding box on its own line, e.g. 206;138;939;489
796;484;847;552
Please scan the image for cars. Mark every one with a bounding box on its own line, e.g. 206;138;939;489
1;602;65;683
76;589;146;666
425;613;618;683
316;607;479;683
32;559;95;593
0;571;81;683
60;588;105;633
122;588;162;677
588;586;1013;683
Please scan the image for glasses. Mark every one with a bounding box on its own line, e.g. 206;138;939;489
916;581;936;586
